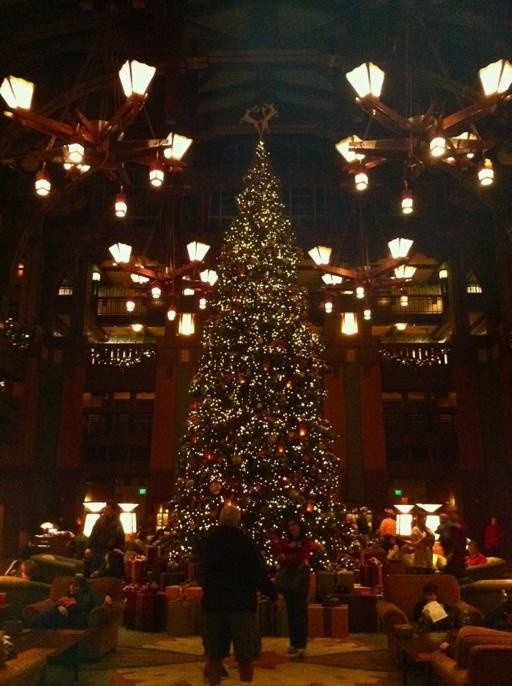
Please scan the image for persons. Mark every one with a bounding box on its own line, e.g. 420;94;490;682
464;540;487;567
375;508;397;540
196;503;283;685
410;509;435;568
385;533;407;561
434;512;450;546
356;506;370;541
414;581;459;629
19;499;165;629
443;504;467;578
439;599;512;659
484;516;505;554
272;515;319;661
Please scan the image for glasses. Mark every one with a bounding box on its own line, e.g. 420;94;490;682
334;58;512;217
307;237;419;337
98;239;220;337
0;57;192;220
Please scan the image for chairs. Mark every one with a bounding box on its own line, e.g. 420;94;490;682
203;646;306;686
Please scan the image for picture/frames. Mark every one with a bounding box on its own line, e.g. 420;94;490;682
0;627;89;686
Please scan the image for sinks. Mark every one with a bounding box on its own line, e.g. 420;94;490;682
363;548;511;686
1;553;122;659
